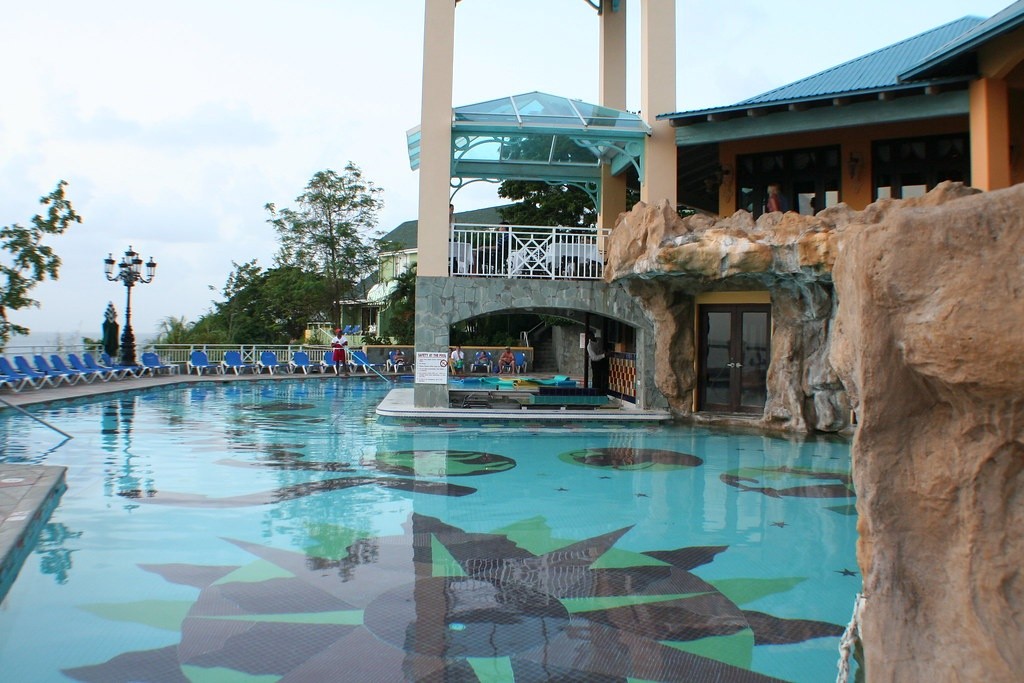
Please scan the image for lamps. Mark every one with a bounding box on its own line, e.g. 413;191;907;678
560;183;569;193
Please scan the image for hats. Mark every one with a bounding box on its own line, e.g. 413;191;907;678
335;329;342;333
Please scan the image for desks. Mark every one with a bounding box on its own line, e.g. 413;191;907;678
515;396;623;410
546;243;603;281
448;242;474;277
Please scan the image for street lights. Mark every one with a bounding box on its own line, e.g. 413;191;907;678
101;243;157;366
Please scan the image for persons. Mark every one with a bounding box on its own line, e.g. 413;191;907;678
393;349;406;366
449;205;458;276
288;336;297;349
562;231;603;277
449;348;452;358
331;328;350;376
472;221;514;273
367;322;378;336
809;196;818;217
766;184;788;215
586;331;610;388
498;346;515;374
450;345;465;373
477;351;489;366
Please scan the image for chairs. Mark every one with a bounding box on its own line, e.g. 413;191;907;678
139;353;181;378
470;351;528;374
256;352;290;376
348;351;370;373
386;351;409;373
289;352;322;375
0;353;142;393
343;324;360;335
220;352;255;376
320;352;344;375
187;350;222;376
